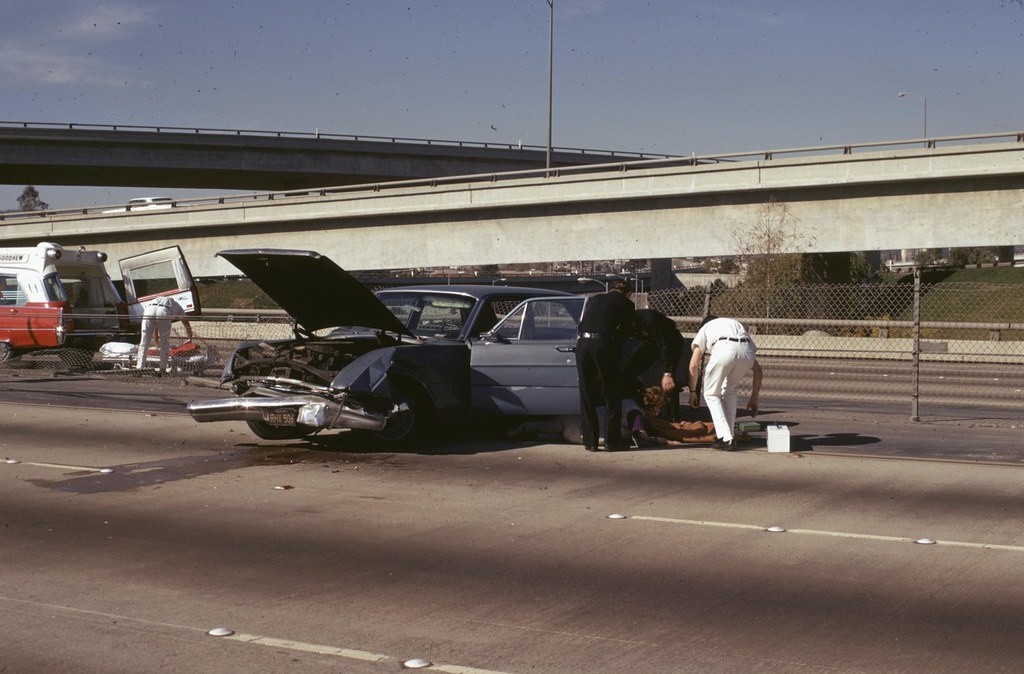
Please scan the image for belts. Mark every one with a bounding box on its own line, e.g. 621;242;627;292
711;337;750;346
147;303;169;310
580;331;607;339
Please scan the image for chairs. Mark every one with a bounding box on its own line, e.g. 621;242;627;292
462;301;499;336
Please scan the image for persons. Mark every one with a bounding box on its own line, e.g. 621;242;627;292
575;281;636;451
621;361;668;446
689;314;763;451
616;309;684;420
132;297;192;377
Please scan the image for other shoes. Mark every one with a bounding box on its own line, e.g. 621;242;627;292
604;439;622;451
584;443;598;452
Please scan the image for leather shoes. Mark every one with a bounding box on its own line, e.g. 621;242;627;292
712;437;735;451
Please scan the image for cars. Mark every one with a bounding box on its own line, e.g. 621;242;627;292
186;248;587;451
0;242;203;367
101;197;172;214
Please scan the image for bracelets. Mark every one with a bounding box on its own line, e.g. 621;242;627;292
690;390;696;392
664;373;671;376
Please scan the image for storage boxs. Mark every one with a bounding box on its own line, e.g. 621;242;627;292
768;421;791;453
739;422;760;431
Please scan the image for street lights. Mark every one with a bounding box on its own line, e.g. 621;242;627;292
448;271;466;285
898;92;927;148
606;273;627;281
630;278;643;293
577;278;608;293
